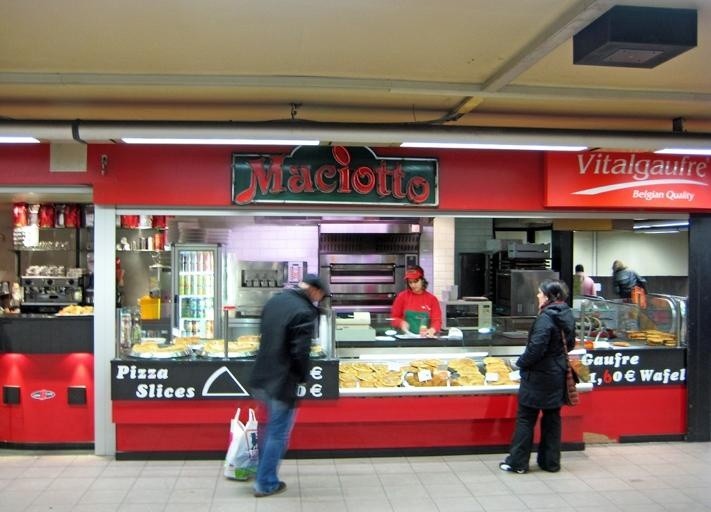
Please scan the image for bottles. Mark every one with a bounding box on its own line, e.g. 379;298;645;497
132;237;154;251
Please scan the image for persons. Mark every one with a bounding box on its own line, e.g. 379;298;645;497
389;264;443;339
243;272;333;499
498;279;577;475
575;264;598;297
612;260;649;298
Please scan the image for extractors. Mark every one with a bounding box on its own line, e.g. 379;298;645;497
318;221;420;252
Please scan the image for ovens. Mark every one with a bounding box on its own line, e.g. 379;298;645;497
320;253;418;313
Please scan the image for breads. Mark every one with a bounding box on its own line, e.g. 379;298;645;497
58;304;93;314
309;345;321;353
627;328;678;347
612;341;631;347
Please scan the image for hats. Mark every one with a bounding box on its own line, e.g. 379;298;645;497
403;265;423;280
302;274;332;297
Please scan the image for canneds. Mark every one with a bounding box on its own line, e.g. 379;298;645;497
178;252;216;338
154;233;164;251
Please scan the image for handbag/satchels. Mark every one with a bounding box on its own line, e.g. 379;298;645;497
224;408;259;480
566;366;580;406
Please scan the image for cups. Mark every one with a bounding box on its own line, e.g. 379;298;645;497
116;237;130;250
23;240;69;250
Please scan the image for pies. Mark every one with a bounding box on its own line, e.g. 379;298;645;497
339;356;520;388
132;334;261;354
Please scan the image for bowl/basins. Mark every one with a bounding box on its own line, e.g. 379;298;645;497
441;285;458;301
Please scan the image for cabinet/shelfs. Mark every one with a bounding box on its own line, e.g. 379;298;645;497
110;307;529;462
9;228;83;313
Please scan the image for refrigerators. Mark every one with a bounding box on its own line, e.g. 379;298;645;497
171;242;221;340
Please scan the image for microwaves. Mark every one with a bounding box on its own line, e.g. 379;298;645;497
439;300;492;331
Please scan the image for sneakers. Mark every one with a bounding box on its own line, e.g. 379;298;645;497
500;463;525;473
255;482;286;497
